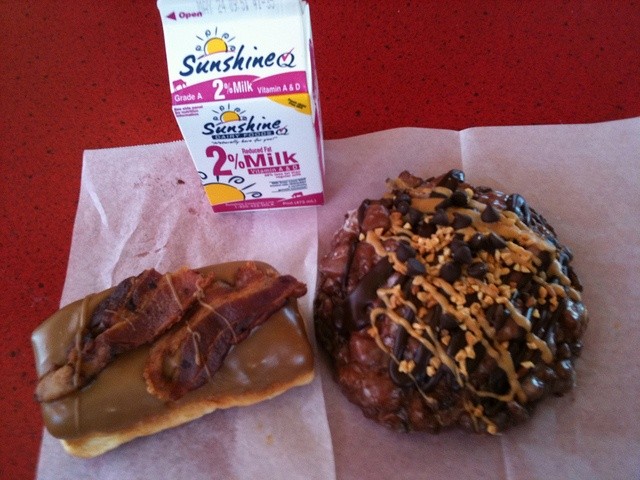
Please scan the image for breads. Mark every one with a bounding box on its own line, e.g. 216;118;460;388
29;259;315;457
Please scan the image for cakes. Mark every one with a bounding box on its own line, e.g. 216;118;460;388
314;166;593;434
